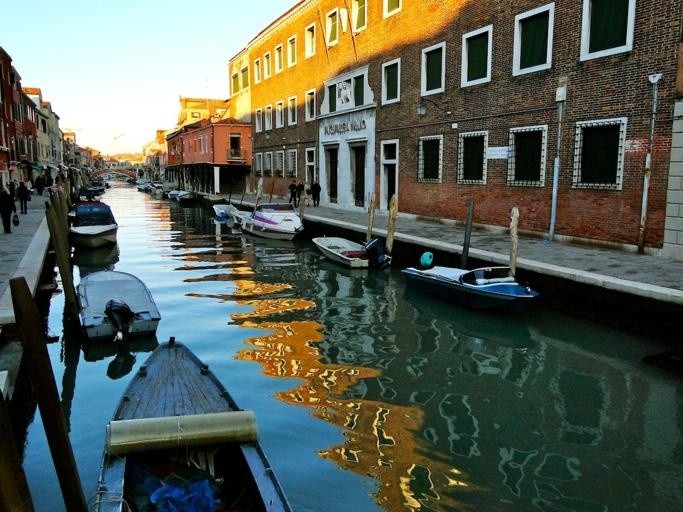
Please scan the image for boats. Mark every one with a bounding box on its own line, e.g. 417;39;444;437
77;269;161;343
137;180;194;205
94;335;292;512
213;204;305;241
399;264;540;310
312;237;392;267
86;185;105;201
68;200;118;248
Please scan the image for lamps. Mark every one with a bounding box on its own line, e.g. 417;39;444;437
416;96;445;117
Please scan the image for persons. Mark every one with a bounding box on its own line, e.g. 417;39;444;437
0;172;66;234
296;180;304;206
304;181;311;207
287;179;297;208
311;181;321;207
255;173;264;204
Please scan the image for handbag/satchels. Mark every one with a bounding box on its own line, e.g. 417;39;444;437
12;214;19;226
